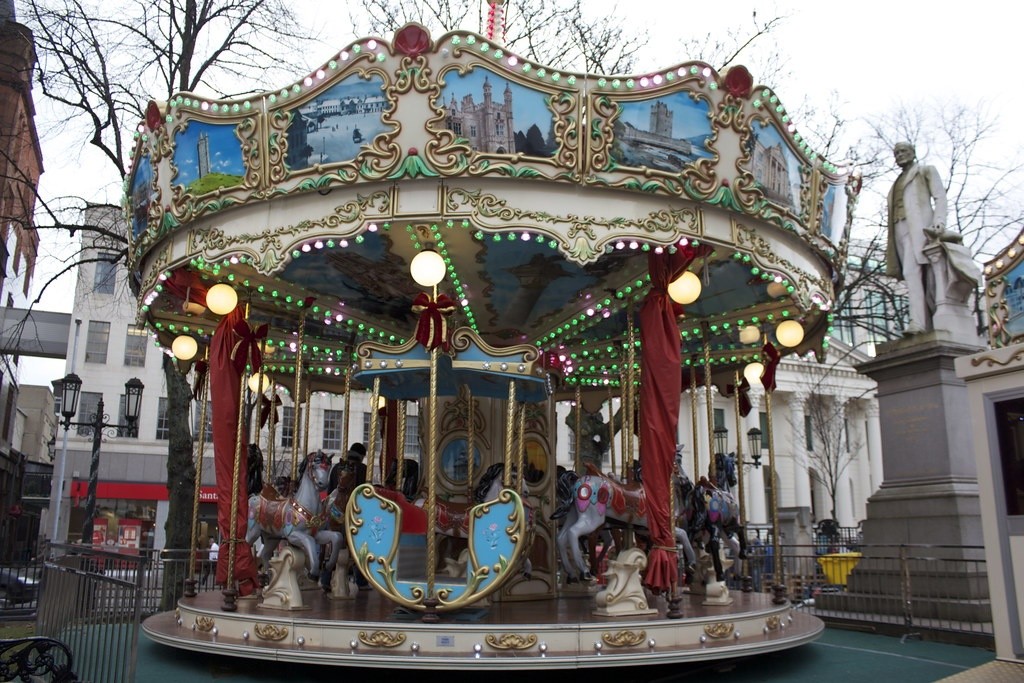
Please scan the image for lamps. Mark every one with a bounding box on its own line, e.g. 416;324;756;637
409;242;447;287
206;282;238;314
667;268;701;304
47;434;56;462
775;317;804;347
171;332;198;361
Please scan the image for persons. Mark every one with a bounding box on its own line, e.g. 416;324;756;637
322;444;371;593
885;143;947;335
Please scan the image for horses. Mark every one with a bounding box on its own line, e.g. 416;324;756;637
550;444;697;585
386;456;419;498
245;449;362;594
690;452;752;582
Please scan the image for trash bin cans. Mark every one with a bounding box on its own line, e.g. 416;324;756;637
44;555;105;627
816;552;863;592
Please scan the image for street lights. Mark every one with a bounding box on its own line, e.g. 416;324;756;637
56;371;145;570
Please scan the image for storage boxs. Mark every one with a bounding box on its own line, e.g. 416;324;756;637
817;552;862;585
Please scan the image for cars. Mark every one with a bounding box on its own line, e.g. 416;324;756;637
0;567;41;608
